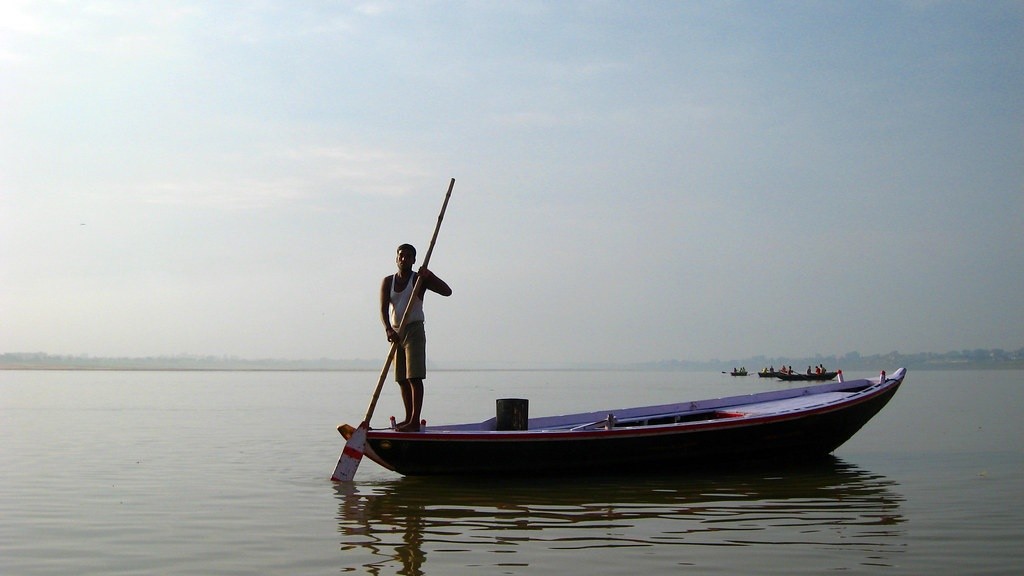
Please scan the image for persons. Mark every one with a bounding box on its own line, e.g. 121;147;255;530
781;365;793;375
379;244;452;430
762;365;774;373
734;367;745;372
807;364;826;374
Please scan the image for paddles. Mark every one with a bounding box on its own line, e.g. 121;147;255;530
331;177;457;482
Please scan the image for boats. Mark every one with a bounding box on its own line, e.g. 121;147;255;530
730;371;748;376
774;372;843;381
339;366;907;479
758;371;783;377
722;371;726;373
